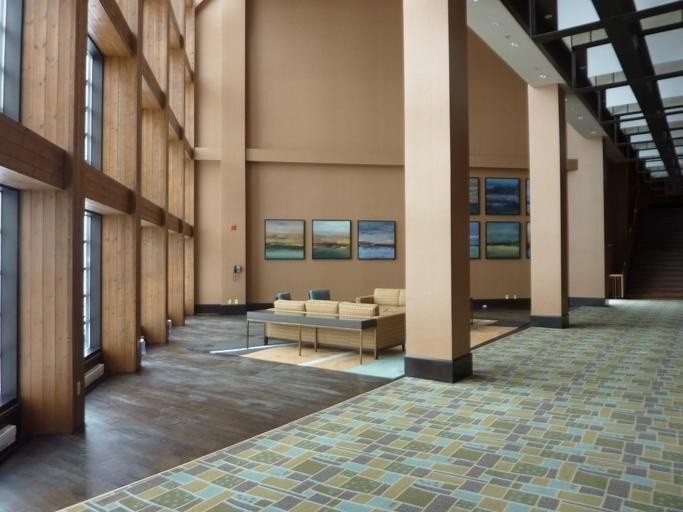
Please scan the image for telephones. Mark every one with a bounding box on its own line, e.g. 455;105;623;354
233;265;240;273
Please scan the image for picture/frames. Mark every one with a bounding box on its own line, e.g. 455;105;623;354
357;220;396;260
312;219;352;260
264;218;305;260
468;176;529;215
469;220;531;260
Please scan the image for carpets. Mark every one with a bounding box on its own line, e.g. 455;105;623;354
207;316;530;380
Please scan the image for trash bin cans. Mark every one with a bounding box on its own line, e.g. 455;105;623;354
607;274;624;299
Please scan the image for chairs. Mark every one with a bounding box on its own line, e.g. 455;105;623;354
246;288;406;367
